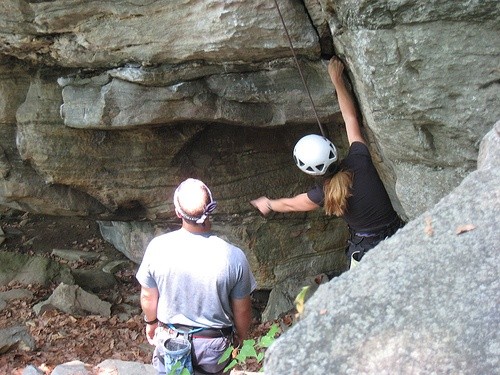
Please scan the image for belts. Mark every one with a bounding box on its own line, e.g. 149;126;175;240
160;323;231;338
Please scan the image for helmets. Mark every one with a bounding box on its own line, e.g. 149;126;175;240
292;134;339;176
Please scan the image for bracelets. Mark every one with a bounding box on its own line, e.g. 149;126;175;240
143;314;159;324
266;199;275;212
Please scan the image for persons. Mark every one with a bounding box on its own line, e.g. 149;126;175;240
247;54;406;272
136;178;260;375
